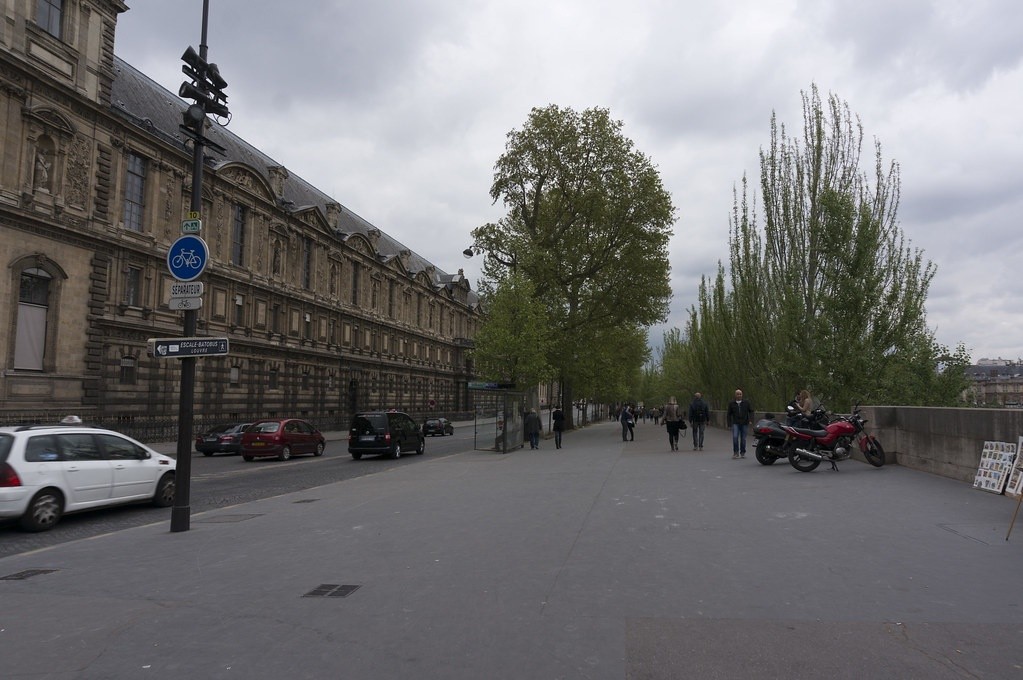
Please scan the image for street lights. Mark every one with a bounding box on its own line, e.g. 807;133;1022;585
170;47;230;531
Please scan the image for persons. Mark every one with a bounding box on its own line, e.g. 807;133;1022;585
552;405;565;449
621;406;635;441
524;408;541;450
495;431;503;449
726;389;752;458
664;396;680;450
689;392;710;451
609;404;663;426
786;384;820;430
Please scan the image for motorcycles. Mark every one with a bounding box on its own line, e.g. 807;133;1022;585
752;401;886;473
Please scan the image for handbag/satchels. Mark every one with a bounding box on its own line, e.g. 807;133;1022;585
678;419;687;429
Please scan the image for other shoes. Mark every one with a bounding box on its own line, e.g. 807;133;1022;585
694;447;698;450
699;447;703;451
535;445;539;449
675;444;678;450
630;439;633;441
741;452;745;457
733;454;739;458
671;445;674;450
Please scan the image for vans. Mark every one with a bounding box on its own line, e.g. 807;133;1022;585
348;411;425;460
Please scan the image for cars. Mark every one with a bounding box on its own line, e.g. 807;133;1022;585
422;418;454;437
240;418;326;461
195;423;249;456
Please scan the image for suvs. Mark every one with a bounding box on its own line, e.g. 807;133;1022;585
0;422;176;531
